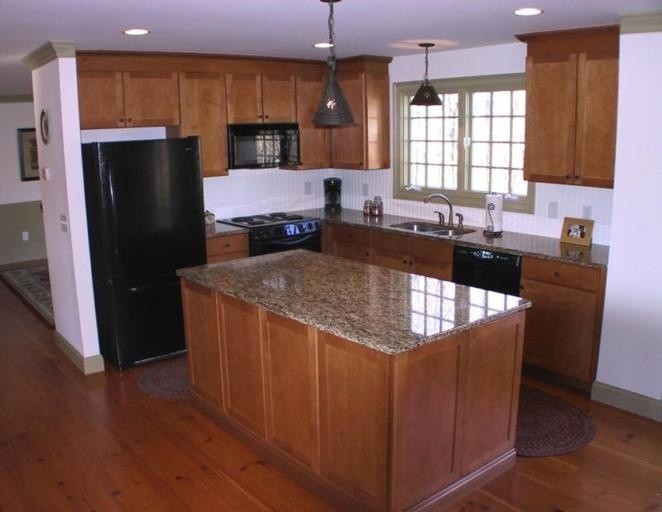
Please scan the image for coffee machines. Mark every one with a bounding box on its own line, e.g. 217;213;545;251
324;177;343;216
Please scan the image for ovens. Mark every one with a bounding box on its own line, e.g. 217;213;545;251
249;231;321;255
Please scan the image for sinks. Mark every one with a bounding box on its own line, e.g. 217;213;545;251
388;220;447;233
433;225;479;236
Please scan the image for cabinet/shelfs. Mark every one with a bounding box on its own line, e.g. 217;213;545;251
516;256;608;383
515;23;619;190
297;58;331;171
330;54;392;172
75;47;181;131
206;233;250;264
370;231;453;283
178;51;228;179
225;53;296;124
330;225;372;265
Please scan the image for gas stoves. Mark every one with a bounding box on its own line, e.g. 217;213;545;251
216;210;324;240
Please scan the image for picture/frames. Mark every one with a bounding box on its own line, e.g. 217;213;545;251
560;216;596;248
15;126;40;183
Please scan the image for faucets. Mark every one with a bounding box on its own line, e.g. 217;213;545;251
423;192;454;229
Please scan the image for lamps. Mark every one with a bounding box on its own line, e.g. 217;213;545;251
311;0;357;128
406;43;445;109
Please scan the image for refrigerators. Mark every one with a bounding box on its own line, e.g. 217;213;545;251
81;132;210;371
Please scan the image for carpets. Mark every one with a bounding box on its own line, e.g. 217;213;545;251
0;263;55;332
134;352;597;458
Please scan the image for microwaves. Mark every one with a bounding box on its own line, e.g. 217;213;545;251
227;123;304;170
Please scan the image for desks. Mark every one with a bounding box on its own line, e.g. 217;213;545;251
174;248;534;512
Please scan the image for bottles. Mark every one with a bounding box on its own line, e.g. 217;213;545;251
363;214;383;227
363;199;372;215
373;195;383;216
